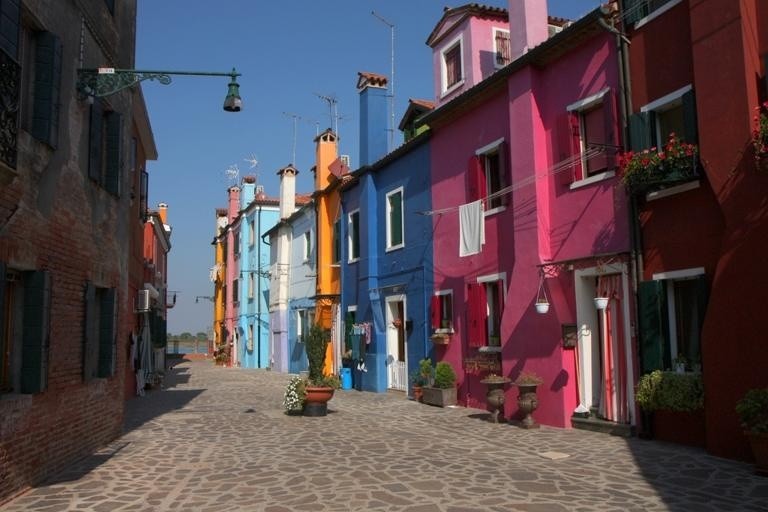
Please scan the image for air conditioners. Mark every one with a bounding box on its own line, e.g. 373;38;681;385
138;289;150;311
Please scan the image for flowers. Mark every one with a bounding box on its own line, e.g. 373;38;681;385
610;128;699;188
282;375;304;415
748;100;768;161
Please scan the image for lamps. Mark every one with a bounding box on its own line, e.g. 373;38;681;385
77;66;243;113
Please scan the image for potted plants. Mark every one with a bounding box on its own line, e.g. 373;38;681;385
634;358;704;443
535;298;550;314
411;356;458;407
297;325;338;402
735;386;767;477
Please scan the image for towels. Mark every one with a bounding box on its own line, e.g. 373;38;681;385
458;199;485;258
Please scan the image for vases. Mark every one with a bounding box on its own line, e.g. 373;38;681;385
594;298;609;309
